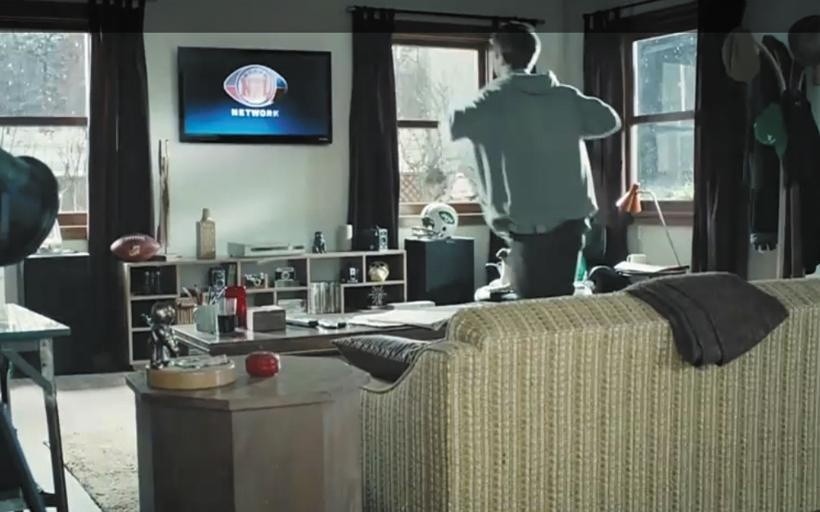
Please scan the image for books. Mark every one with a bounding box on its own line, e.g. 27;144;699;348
177;302;194;325
308;281;340;314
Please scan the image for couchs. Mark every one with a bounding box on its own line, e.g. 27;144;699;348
360;280;817;509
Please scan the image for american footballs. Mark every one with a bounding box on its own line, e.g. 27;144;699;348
110;235;160;261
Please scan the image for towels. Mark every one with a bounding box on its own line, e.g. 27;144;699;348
621;269;788;373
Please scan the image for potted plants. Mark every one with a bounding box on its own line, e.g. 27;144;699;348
365;287;390;310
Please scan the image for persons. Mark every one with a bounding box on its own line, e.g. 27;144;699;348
437;19;622;304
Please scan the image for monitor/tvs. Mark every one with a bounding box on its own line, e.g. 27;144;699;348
176;45;334;145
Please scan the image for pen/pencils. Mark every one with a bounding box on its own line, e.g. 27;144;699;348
180;285;227;306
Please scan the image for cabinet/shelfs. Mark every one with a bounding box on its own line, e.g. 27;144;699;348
110;249;408;369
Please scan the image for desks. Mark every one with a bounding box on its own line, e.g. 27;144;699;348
2;295;70;512
124;356;369;511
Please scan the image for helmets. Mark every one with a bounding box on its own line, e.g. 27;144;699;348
412;202;458;240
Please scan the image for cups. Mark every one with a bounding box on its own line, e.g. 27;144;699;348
216;297;237;336
195;207;217;260
626;253;646;265
192;304;216;335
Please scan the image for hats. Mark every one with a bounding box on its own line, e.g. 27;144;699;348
754;102;788;158
721;27;760;82
789;14;819;64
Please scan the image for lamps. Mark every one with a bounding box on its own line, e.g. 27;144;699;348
613;182;682;267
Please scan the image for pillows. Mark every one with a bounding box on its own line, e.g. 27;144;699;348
329;332;429;382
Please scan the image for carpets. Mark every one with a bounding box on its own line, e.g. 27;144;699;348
42;428;142;512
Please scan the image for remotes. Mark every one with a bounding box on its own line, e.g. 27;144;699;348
286;315;319;328
